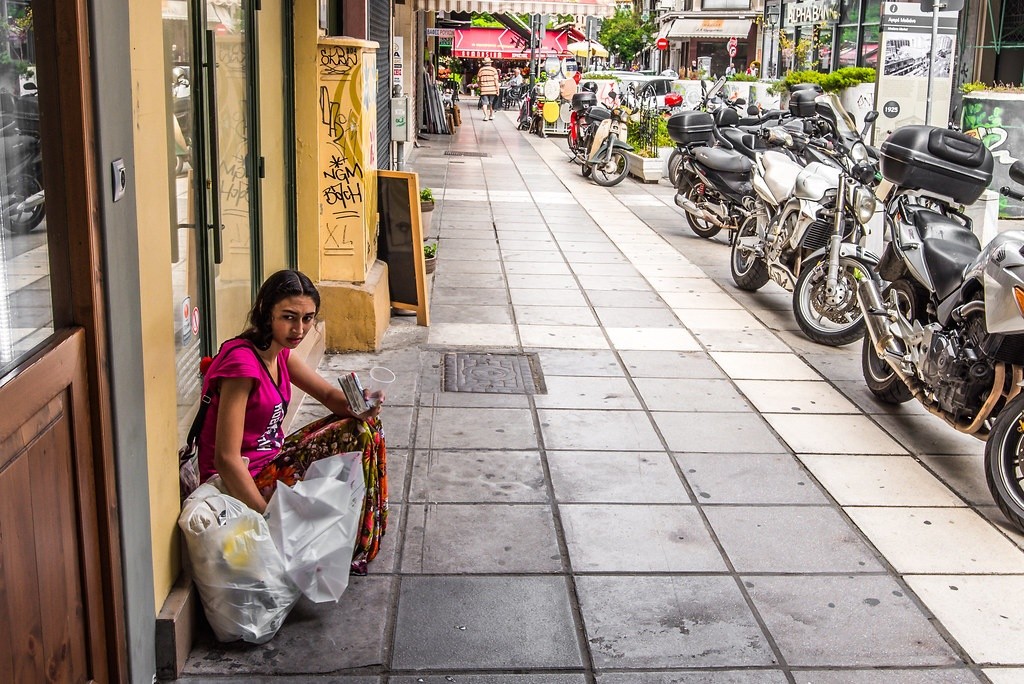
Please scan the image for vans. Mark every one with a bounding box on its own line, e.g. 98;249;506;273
618;75;676;108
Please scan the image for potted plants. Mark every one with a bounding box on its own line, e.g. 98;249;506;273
627;141;663;183
822;66;876;146
578;73;622;109
424;243;437;275
671;79;703;110
720;73;754;119
751;78;781;111
420;187;434;212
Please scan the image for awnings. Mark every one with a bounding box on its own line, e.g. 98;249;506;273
450;27;568;59
651;18;753;45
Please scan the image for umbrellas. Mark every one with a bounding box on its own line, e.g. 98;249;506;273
568;41;609;70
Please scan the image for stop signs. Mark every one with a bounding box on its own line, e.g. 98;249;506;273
658;38;668;49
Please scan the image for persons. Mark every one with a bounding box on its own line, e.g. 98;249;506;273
493;66;528;110
746;63;760;79
195;269;390;581
725;63;735;79
688;60;698;79
477;56;500;121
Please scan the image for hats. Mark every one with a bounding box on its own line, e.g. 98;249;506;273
482;57;493;63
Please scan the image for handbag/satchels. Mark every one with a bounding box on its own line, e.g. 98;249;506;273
178;445;201;490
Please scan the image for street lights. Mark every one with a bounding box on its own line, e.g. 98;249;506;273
642;35;647;70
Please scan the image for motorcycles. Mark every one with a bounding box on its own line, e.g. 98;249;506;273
659;75;880;238
568;91;640;187
730;141;886;345
861;125;1024;532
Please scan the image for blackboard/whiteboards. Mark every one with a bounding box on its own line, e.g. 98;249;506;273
378;168;429;327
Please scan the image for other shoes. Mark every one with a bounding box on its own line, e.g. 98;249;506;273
489;116;494;119
484;117;488;120
